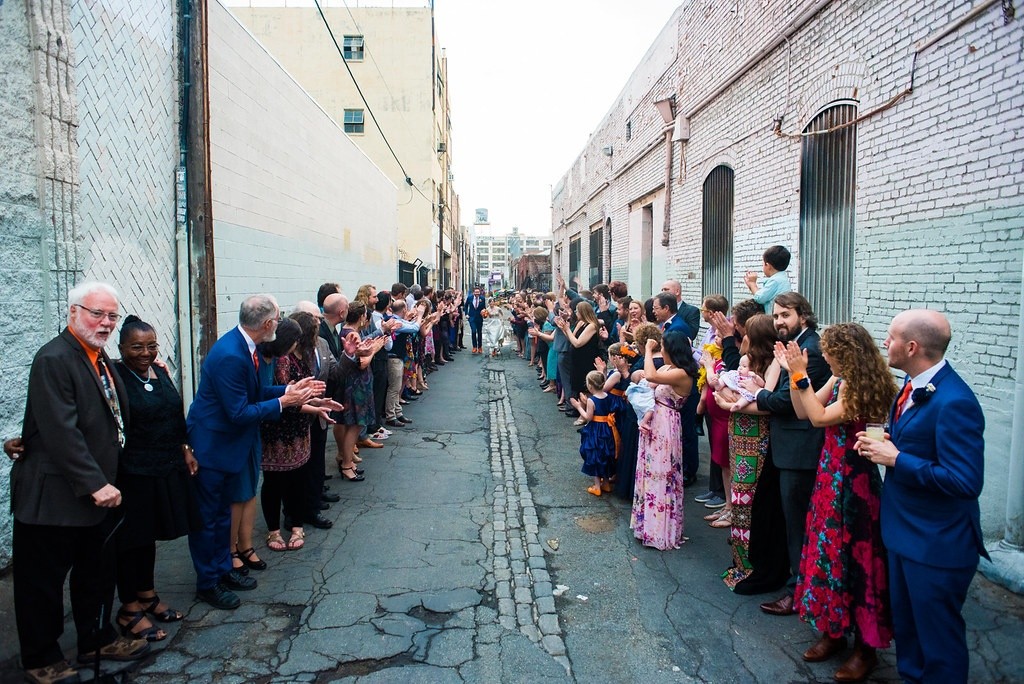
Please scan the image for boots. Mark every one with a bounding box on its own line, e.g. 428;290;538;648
834;633;878;683
801;631;849;662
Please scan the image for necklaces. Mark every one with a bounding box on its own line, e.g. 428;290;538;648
119;359;154;393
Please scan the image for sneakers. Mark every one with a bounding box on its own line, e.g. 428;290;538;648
695;491;727;508
77;635;152;664
25;661;82;684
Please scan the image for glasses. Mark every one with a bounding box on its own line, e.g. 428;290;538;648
698;307;710;314
73;303;122;323
263;316;283;324
122;343;160;352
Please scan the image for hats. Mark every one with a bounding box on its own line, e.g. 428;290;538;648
293;300;324;318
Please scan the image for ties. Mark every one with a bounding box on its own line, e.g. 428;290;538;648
97;351;126;449
894;381;913;423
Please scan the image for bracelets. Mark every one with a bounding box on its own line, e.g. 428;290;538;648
182;445;194;455
788;372;811;393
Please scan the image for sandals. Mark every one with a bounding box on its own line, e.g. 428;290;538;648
265;530;306;551
703;507;733;528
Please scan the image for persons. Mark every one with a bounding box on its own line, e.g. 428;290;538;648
744;245;793;326
494;270;794;529
8;278;151;684
316;284;465;482
483;297;505;356
773;323;903;684
229;315;304;576
290;300;360;512
630;329;702;552
3;313;200;642
711;313;780;590
184;294;327;610
464;287;488;353
261;313;344;552
853;308;993;683
733;290;834;616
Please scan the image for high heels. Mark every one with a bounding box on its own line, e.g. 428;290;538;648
115;602;167;642
138;594;183;624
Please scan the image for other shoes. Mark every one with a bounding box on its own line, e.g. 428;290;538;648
318;342;468;509
587;483;612;496
683;466;697;488
471;345;590;429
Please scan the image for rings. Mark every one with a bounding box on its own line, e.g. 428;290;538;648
313;389;315;392
12;452;18;460
865;451;868;457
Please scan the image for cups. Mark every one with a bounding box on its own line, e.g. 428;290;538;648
866;424;885;442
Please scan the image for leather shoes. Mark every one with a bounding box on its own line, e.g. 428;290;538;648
196;570;258;610
230;543;267;576
284;513;333;532
760;594;799;616
